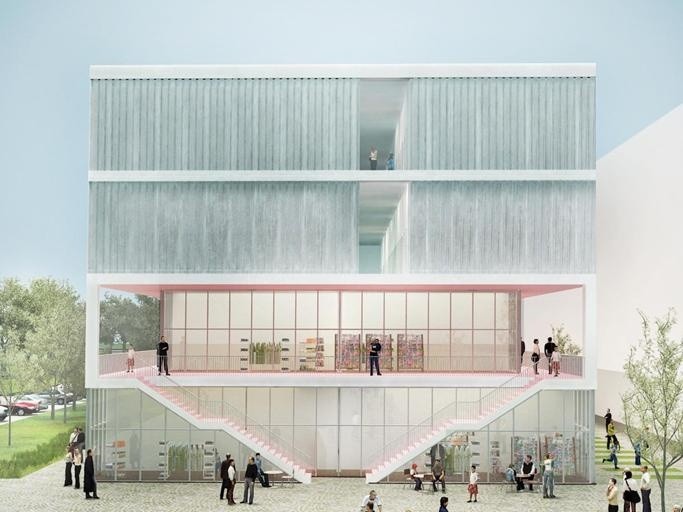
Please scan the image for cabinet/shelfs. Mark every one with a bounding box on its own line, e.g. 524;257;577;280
241;334;423;372
105;440;216;481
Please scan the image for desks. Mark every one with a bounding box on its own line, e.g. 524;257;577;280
414;473;434;490
264;471;283;489
515;474;530;492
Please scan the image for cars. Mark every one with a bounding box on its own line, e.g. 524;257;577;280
0;386;75;423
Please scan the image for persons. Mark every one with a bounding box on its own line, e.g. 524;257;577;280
218;450;271;506
431;458;445;492
154;334;172;377
505;453;555;498
635;441;642;465
607;466;652;512
385;152;395;170
63;427;99;500
439;497;449;512
467;465;479;503
521;335;561;377
126;343;136;374
410;464;422;491
603;408;620;470
369;145;378;169
361;490;382;510
368;339;383;376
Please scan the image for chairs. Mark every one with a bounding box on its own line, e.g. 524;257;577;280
503;481;517;493
527;473;555;491
520;468;537;481
434;471;445;490
422;475;433;490
282;469;294;488
404;469;414;488
